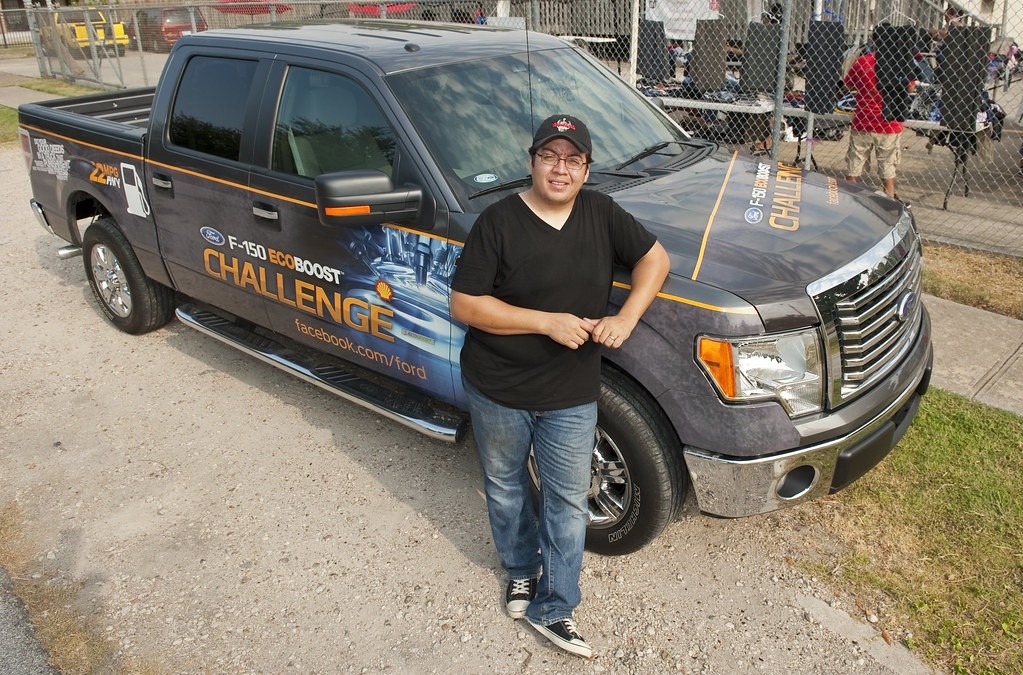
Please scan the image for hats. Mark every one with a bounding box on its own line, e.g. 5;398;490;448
530;114;592;158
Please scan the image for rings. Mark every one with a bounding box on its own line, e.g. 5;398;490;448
608;336;615;341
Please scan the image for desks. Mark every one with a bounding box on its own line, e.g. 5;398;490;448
553;36;1007;213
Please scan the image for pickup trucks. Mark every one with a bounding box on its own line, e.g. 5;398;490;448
40;6;128;60
17;17;939;556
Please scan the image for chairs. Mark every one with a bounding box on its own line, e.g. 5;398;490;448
287;88;392;184
406;71;533;171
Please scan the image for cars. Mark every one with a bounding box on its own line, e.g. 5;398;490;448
125;5;209;52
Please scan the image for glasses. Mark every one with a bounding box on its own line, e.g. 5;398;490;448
535;152;586;170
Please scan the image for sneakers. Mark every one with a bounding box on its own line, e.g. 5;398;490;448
506;578;537;618
526;615;592;659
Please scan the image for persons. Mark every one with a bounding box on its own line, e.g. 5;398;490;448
450;115;671;659
845;24;916;198
1003;44;1021;93
